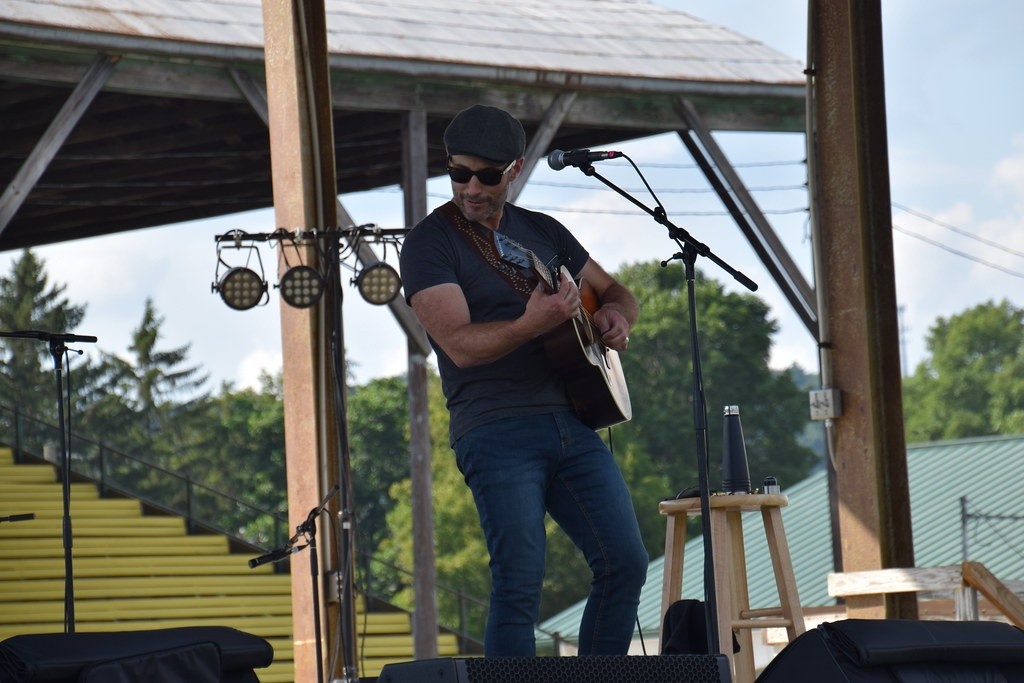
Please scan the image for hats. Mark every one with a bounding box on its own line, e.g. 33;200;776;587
443;105;526;163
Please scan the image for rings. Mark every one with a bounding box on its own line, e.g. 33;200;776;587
625;336;630;342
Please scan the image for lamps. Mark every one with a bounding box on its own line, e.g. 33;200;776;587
350;235;403;305
273;239;325;309
211;240;269;311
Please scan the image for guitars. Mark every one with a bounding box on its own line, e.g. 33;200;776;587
489;228;634;434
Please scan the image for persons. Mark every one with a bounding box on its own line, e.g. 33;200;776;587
396;101;648;658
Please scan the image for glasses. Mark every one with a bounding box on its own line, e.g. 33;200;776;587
447;155;517;186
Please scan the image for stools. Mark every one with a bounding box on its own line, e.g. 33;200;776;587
658;494;807;683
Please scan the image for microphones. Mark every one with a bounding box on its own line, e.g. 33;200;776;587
248;545;307;568
548;149;623;170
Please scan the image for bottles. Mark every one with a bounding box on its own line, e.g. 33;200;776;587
721;405;753;494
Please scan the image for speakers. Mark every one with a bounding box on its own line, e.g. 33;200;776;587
752;620;1024;683
378;652;734;683
